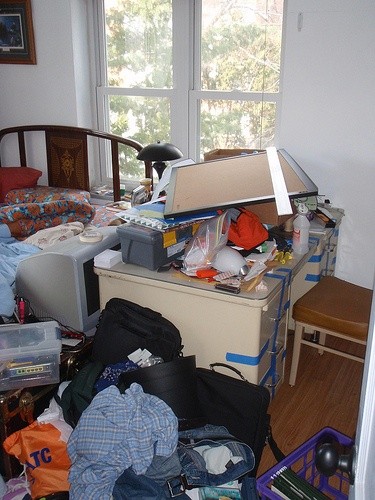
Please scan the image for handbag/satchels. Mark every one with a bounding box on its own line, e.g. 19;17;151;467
195;362;271;476
93;295;183;359
3;417;75;500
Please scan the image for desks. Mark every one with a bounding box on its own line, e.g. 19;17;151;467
204;150;297;225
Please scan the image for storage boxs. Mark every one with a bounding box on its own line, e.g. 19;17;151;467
256;427;354;500
117;223;200;271
0;322;61;391
0;338;93;483
94;249;122;268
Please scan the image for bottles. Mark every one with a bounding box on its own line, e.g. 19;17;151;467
293;204;311;255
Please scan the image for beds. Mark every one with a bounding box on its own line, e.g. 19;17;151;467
0;125;153;320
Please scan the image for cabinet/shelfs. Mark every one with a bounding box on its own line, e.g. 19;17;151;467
94;209;344;404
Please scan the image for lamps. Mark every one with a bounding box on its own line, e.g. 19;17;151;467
136;140;184;180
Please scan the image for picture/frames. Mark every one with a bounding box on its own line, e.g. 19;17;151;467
0;0;36;65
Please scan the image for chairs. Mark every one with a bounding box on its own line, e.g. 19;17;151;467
288;275;373;387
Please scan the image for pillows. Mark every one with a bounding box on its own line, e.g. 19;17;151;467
0;166;42;203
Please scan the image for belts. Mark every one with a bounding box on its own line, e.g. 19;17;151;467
166;474;200;498
